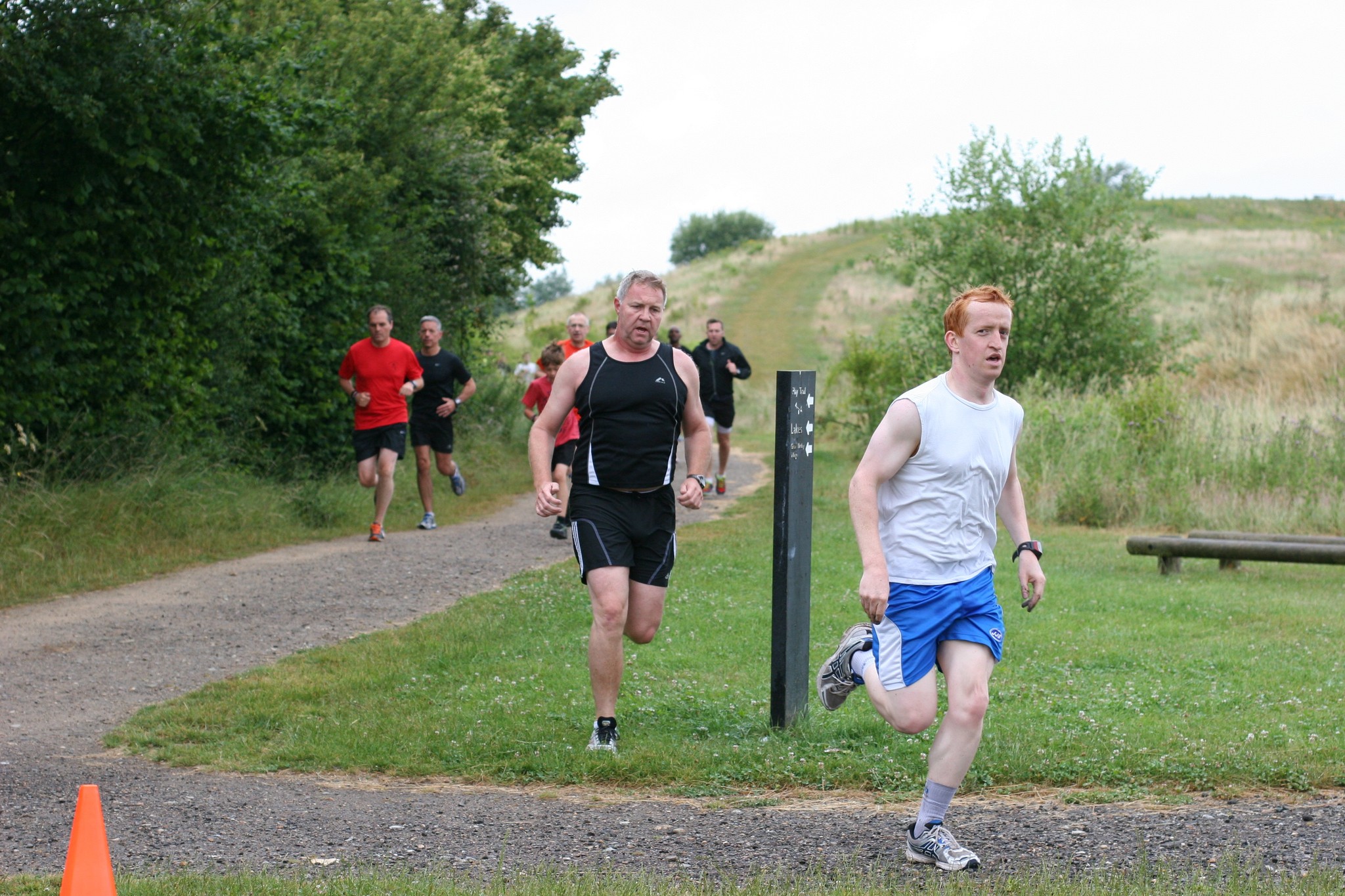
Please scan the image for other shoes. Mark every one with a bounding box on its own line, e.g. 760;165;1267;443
701;481;712;493
715;472;726;494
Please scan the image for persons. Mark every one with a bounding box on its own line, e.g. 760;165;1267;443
692;319;752;496
667;328;693;442
535;312;595;480
339;305;424;541
605;321;618;338
818;286;1046;870
403;316;476;530
521;343;581;540
527;269;711;754
515;352;541;390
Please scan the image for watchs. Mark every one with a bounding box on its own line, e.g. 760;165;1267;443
1012;540;1043;563
686;474;706;489
454;398;462;408
736;369;741;375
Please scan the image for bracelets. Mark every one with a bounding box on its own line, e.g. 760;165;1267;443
351;390;357;398
409;380;417;391
531;415;536;422
354;392;359;399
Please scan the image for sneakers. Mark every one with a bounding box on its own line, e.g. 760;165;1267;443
448;465;464;495
373;487;378;505
417;514;436;529
817;623;873;710
586;716;620;753
550;521;567;539
906;820;980;872
369;521;385;541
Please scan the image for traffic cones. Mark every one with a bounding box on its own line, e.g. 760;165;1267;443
52;784;116;895
